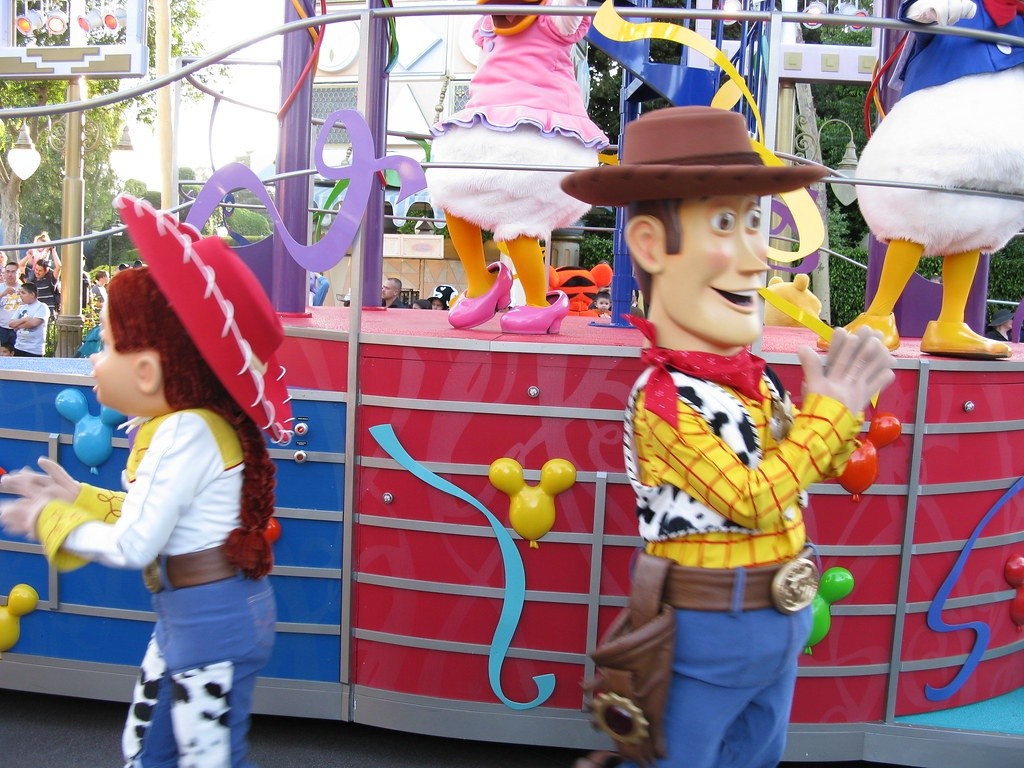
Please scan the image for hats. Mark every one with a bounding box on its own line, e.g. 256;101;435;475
428;285;458;309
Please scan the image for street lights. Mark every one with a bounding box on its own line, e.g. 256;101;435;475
764;118;859;322
4;102;140;361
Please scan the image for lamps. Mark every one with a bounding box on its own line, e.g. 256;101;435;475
20;6;126;34
717;0;868;31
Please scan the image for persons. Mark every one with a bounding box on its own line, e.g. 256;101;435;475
0;192;293;768
560;107;898;768
82;256;109;313
309;272;331;306
986;309;1014;341
382;278;459;310
0;232;63;357
594;290;639;318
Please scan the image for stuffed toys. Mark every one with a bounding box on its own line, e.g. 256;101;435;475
424;0;612;335
817;0;1024;358
